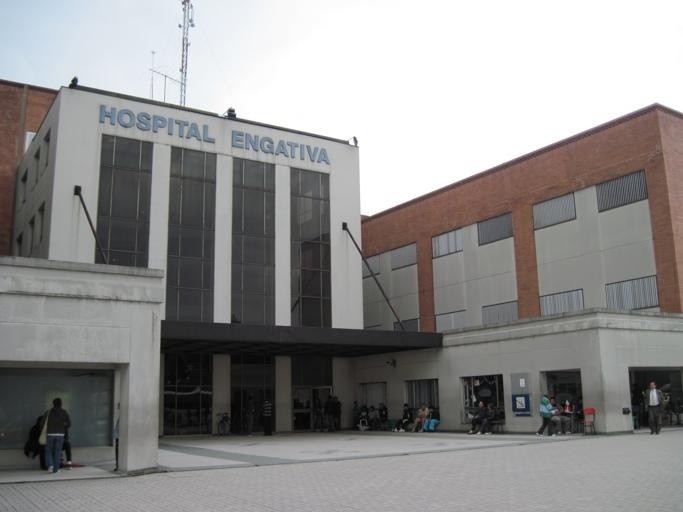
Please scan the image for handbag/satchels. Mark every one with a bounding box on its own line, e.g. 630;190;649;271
38;424;47;445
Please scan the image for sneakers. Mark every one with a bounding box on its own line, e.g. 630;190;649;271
47;461;72;474
469;430;492;435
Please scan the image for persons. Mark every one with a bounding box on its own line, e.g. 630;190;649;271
261;400;273;435
114;403;120;471
398;402;440;432
314;396;341;432
469;400;496;435
352;401;388;431
30;398;72;474
248;395;256;435
631;381;662;434
536;392;583;437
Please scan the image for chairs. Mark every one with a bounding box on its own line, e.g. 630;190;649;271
581;407;596;436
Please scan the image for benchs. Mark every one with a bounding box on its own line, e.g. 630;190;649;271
461;421;505;434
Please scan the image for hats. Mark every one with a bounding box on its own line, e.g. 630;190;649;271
543;391;551;399
